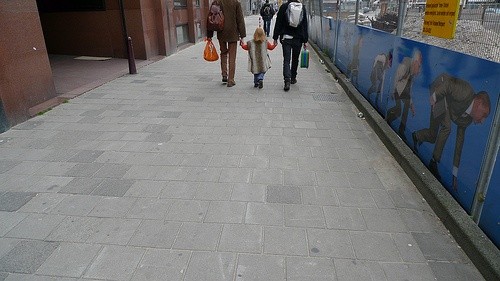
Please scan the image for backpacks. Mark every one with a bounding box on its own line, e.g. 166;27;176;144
287;2;303;27
263;4;271;16
207;0;224;31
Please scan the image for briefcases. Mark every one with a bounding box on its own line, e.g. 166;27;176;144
301;47;309;68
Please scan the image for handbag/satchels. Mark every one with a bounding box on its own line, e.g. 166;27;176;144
203;38;219;62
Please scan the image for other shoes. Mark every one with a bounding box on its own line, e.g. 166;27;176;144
254;83;259;87
284;82;290;91
227;80;235;87
291;78;297;84
222;78;227;82
259;80;263;89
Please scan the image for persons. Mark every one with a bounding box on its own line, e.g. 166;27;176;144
275;4;278;12
366;49;393;108
408;72;491;194
272;0;309;92
260;0;275;37
207;0;247;87
240;27;278;89
387;48;422;136
